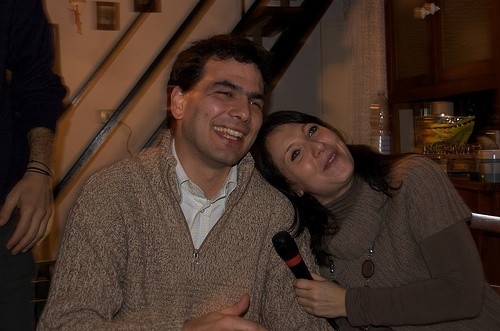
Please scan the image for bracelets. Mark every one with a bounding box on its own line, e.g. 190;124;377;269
25;160;54;177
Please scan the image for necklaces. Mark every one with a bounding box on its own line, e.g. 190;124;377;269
327;244;375;331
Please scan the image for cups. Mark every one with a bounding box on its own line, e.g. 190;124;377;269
473;149;500;183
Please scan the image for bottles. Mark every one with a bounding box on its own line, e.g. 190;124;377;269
369;91;392;156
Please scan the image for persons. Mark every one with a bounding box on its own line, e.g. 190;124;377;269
250;110;500;331
0;0;78;331
36;35;340;331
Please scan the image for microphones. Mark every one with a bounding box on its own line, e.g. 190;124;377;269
272;231;339;330
